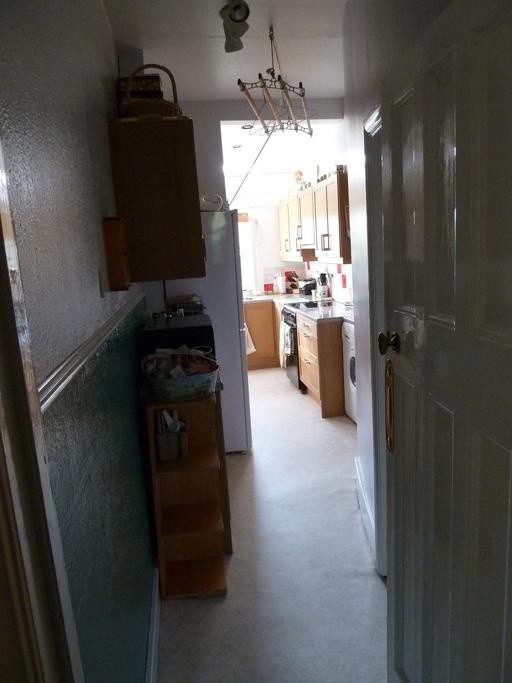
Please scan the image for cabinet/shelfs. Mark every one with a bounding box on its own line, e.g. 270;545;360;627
143;397;228;600
314;174;351;266
278;187;317;261
295;313;345;418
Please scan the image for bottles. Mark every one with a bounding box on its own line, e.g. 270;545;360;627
274;272;286;294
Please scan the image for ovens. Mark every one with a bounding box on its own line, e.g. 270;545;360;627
281;306;306;392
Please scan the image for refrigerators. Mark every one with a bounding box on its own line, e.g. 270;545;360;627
164;209;252;456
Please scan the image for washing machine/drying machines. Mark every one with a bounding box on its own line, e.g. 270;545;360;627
340;321;358;424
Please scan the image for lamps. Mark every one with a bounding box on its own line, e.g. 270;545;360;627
218;0;250;53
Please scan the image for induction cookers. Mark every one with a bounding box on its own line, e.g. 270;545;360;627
289;301;333;309
